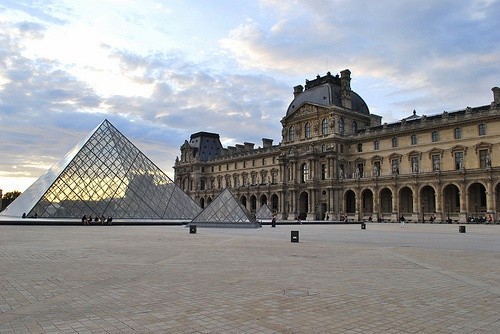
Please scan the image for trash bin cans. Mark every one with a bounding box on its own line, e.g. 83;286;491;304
189;223;199;235
360;223;367;230
290;230;300;243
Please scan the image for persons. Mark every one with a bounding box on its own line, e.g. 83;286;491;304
467;215;492;224
297;215;302;225
344;216;348;222
369;215;373;223
400;215;405;228
82;215;113;226
254;215;256;221
22;212;37;218
429;214;436;223
271;215;278;227
